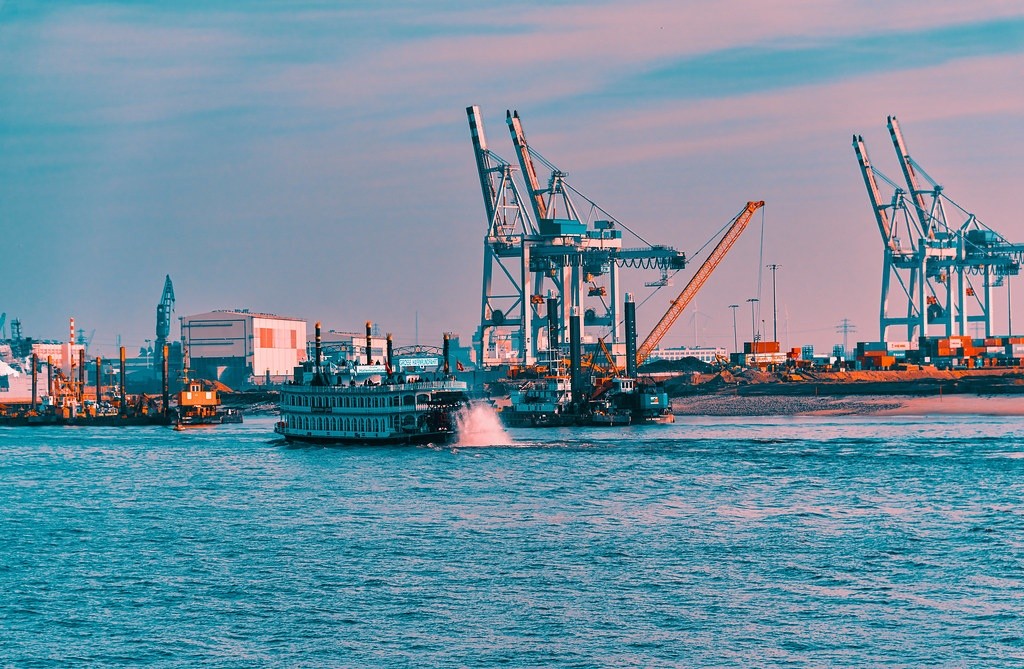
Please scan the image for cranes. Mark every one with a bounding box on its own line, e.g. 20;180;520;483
849;112;1024;364
461;101;688;380
151;273;175;365
514;198;769;394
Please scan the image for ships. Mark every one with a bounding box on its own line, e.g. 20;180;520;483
273;363;472;446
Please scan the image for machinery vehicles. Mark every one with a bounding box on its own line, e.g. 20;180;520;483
709;353;729;367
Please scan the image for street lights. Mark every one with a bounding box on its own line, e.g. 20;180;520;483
728;305;741;353
745;298;760;342
766;264;784;341
